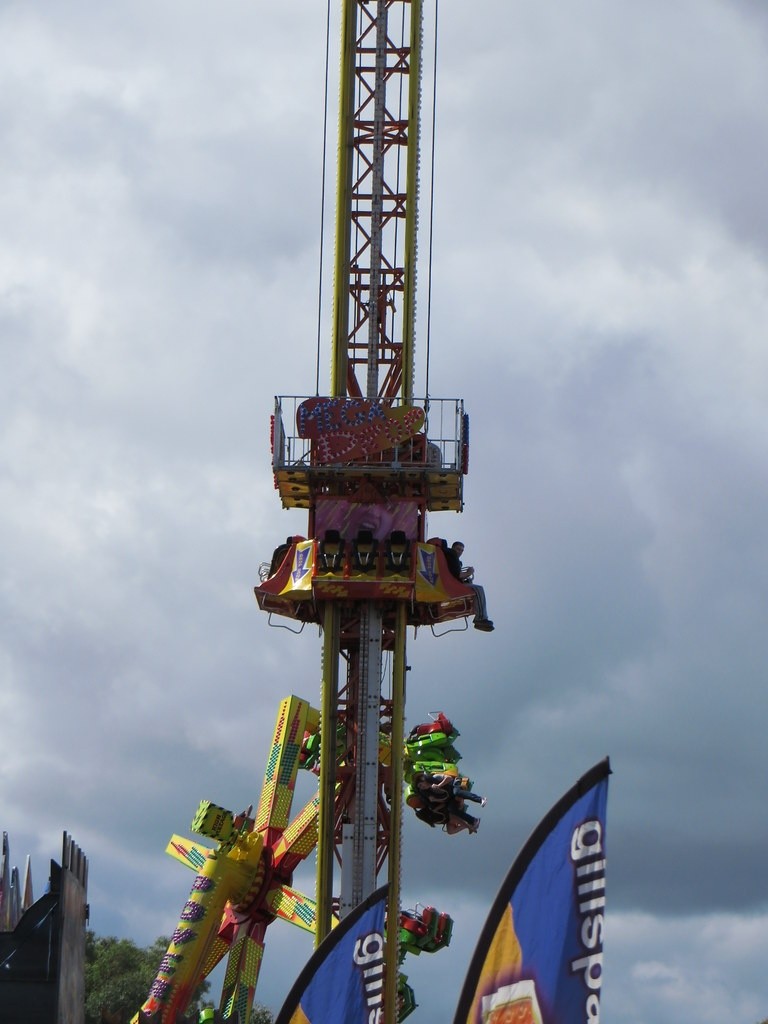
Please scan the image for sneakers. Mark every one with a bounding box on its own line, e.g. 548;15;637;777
474;617;494;632
475;818;480;830
481;797;488;807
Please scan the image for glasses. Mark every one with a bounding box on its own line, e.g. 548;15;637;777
454;546;463;551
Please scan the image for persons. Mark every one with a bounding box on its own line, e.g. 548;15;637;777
452;542;494;631
417;775;485;830
